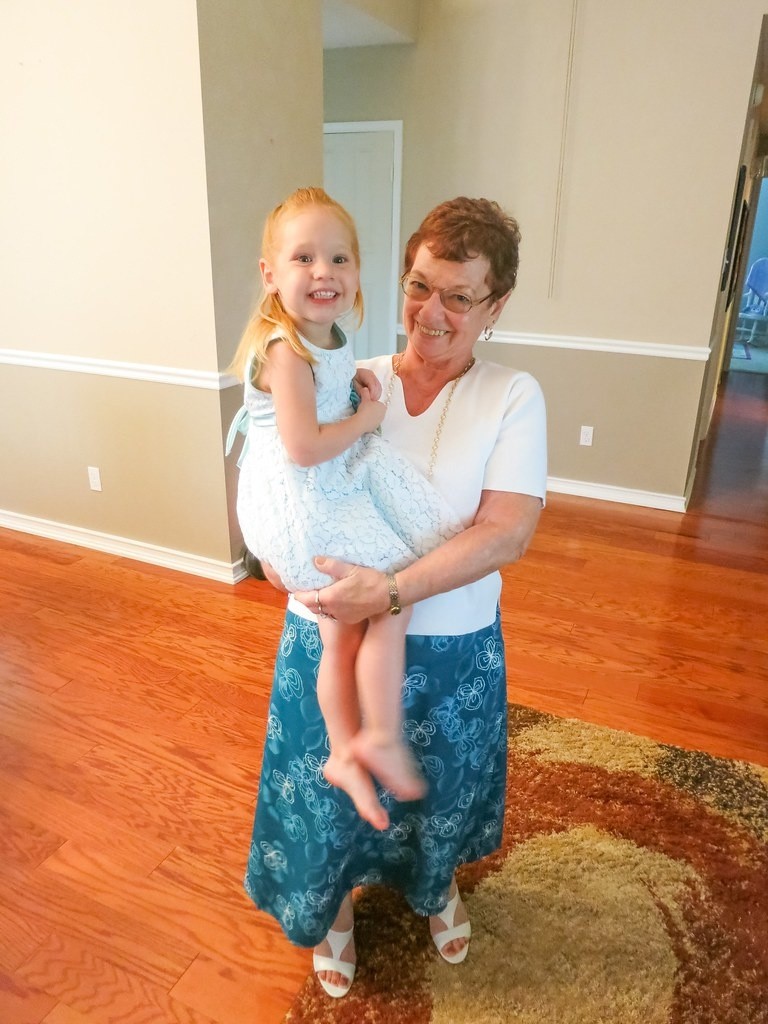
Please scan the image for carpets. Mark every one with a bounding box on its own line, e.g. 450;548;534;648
729;343;768;375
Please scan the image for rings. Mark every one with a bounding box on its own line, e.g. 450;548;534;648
319;605;327;618
330;615;337;622
316;590;321;604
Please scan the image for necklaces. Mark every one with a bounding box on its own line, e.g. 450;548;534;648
385;351;475;481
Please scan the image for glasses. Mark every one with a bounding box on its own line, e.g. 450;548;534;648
399;269;496;313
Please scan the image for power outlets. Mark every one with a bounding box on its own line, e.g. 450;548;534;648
88;466;102;492
580;426;594;447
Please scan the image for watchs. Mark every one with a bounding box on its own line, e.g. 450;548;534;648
389;575;401;616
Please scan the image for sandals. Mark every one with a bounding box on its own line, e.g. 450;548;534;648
431;884;471;963
313;927;356;998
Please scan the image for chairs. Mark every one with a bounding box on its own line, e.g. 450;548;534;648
734;258;768;343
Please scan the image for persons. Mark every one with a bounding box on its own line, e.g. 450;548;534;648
245;198;550;1000
224;186;429;832
